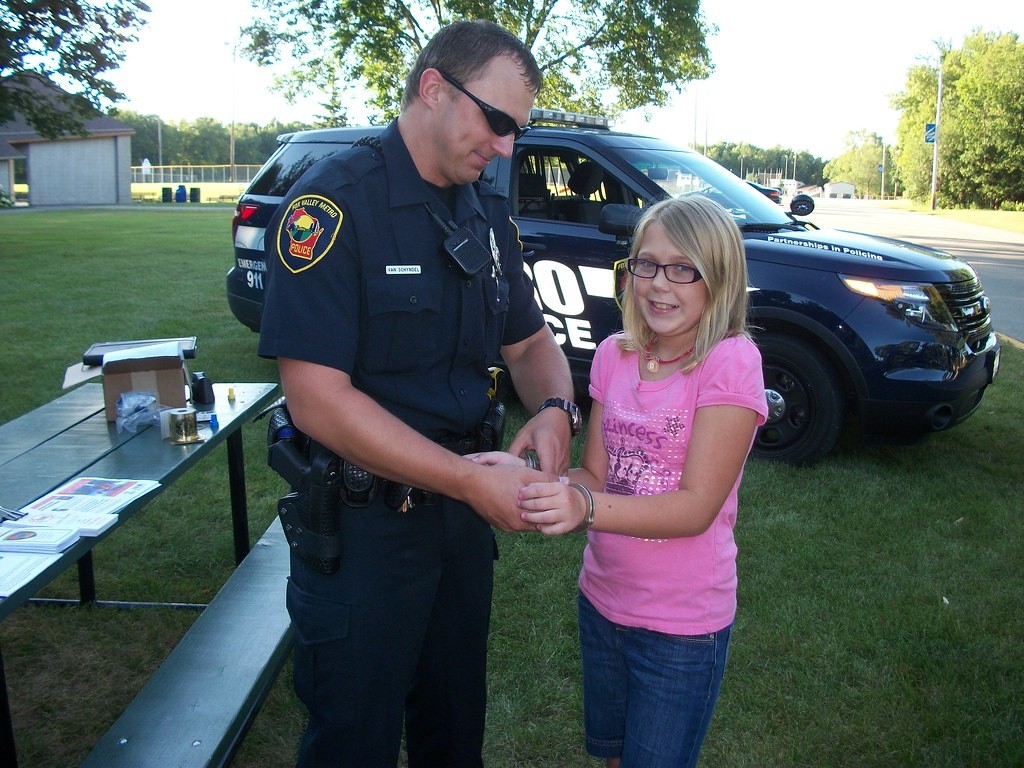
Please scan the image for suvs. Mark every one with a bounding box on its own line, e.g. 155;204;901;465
225;109;1000;468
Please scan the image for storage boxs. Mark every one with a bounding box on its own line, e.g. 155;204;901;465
60;350;192;424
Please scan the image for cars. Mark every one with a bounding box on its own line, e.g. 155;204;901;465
680;180;781;208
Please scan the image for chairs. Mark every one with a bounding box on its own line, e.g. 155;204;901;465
568;161;603;225
517;173;556;220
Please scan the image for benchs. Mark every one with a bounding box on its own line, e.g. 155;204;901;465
79;515;300;768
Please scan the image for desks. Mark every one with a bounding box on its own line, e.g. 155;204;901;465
0;380;280;768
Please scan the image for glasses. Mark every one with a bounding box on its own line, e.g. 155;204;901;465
440;68;531;144
625;258;703;285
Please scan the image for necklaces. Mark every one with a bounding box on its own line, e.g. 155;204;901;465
645;335;695;372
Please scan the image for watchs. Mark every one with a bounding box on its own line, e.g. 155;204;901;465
538;397;582;438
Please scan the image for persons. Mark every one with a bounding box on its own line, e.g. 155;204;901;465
142;159;151;183
256;21;576;768
465;196;768;768
